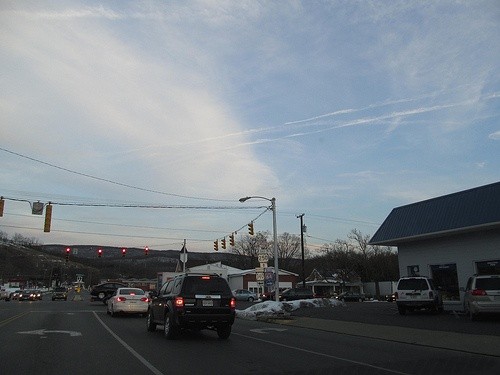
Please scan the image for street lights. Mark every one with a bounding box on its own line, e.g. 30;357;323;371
238;195;279;302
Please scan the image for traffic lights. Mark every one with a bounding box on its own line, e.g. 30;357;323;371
213;240;218;251
123;249;126;256
248;223;253;235
65;248;70;262
98;250;102;259
145;247;148;256
229;235;234;246
221;239;226;249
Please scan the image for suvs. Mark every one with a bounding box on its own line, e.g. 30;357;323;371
147;273;235;339
52;287;68;301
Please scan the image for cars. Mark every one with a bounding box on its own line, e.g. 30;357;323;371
331;292;363;303
273;288;315;301
260;288;285;301
90;283;128;305
460;275;500;320
394;276;443;314
107;288;149;317
232;289;255;301
0;288;43;302
